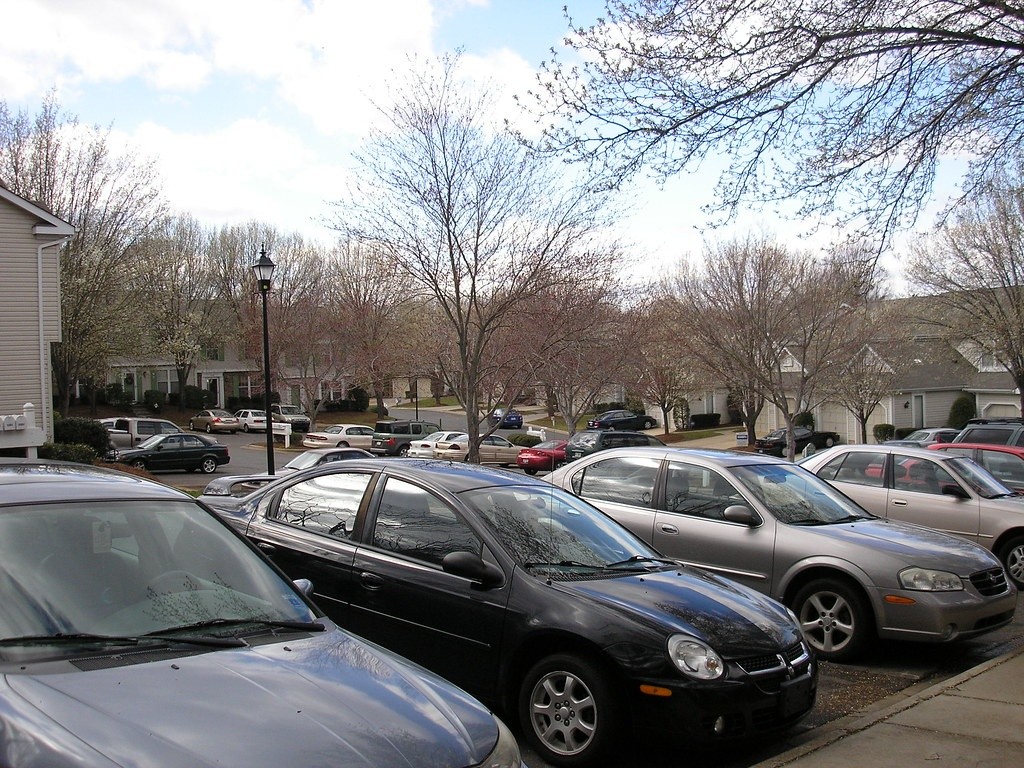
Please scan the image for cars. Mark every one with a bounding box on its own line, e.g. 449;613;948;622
490;408;523;430
862;418;1024;495
516;438;569;475
433;433;530;468
302;424;375;450
0;454;533;768
586;408;657;431
240;447;376;492
489;446;1018;665
168;455;819;768
104;433;230;475
759;444;1024;593
95;417;121;430
753;425;840;457
188;409;240;434
406;431;467;459
233;409;267;433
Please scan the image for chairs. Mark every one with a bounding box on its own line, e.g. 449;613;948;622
170;439;174;444
999;462;1010;481
668;475;697;517
35;516;145;634
379;488;435;560
893;465;930;493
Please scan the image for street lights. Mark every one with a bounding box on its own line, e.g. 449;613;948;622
250;243;275;474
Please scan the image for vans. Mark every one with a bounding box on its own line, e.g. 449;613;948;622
369;419;442;457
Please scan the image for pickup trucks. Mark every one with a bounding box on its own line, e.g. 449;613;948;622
107;418;218;451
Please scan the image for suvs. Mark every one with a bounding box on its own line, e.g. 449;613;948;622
271;403;310;433
566;427;668;463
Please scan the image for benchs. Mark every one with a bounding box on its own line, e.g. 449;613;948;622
819;467;878;485
